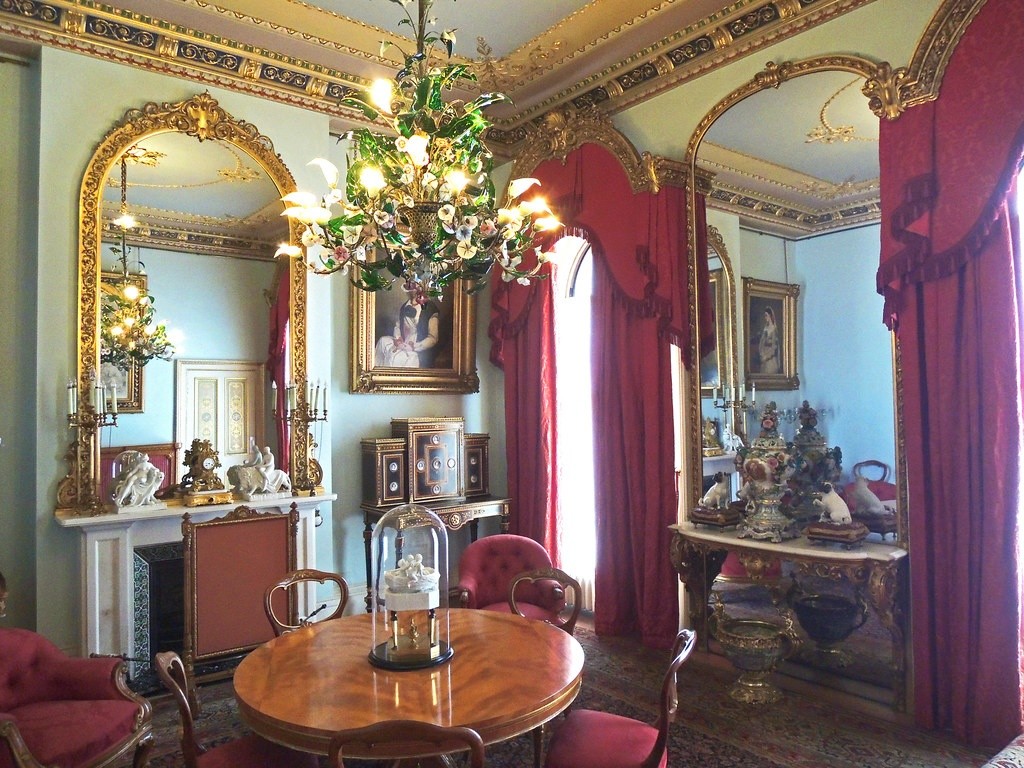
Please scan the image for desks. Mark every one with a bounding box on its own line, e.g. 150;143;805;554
359;492;514;614
667;520;913;713
233;607;584;768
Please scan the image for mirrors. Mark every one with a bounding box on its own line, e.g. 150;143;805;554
53;87;324;518
702;223;739;398
684;54;884;550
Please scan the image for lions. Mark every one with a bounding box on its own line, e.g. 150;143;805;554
226;465;242;493
235;466;292;496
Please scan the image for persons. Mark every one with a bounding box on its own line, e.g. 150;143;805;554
118;452;159;505
243;444;262;467
398;553;424;588
255;446;275;492
115;452;143;478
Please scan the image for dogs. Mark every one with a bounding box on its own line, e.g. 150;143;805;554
811;481;853;526
697;470;729;509
848;477;885;515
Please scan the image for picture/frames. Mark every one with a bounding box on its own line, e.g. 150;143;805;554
740;277;801;392
347;238;480;395
98;271;145;413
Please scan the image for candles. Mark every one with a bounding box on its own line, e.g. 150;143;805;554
711;380;755;408
65;368;118;426
270;373;328;422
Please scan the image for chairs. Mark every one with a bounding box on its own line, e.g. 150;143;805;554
505;567;583;637
543;629;697;768
329;716;484;768
264;569;349;637
154;650;321;768
457;533;567;628
1;627;155;768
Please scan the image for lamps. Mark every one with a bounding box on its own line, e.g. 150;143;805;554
101;145;167;236
99;145;175;372
270;0;567;301
